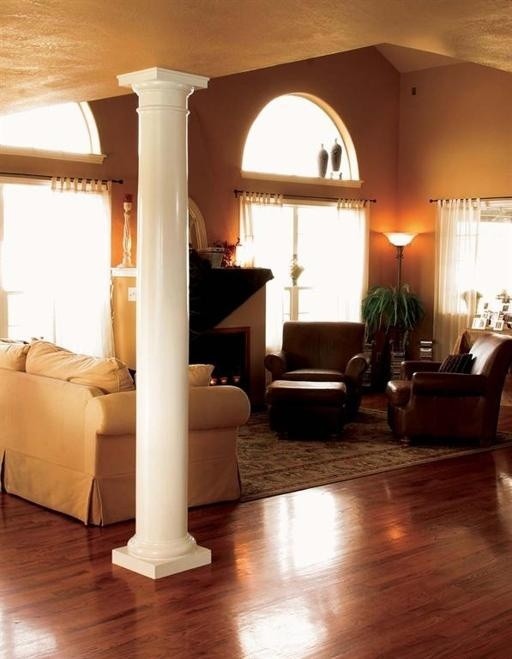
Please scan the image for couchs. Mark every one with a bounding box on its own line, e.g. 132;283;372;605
3;340;246;524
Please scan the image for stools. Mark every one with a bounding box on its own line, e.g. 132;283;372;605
264;378;348;439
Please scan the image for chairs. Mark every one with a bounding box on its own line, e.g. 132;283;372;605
261;322;370;422
383;328;512;451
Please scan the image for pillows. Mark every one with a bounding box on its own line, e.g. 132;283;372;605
440;352;471;374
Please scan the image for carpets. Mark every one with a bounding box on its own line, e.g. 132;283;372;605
239;405;510;504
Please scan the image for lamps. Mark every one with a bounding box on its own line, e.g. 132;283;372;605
383;228;419;286
